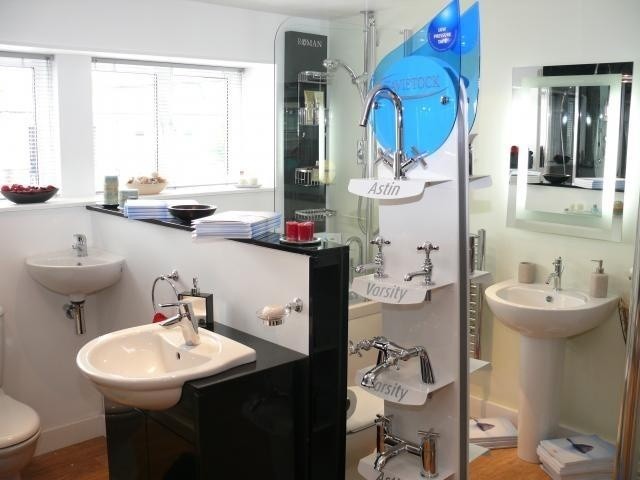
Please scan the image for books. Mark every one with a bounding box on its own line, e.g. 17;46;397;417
536;435;616;480
469;417;518;450
125;199;201;219
192;209;281;239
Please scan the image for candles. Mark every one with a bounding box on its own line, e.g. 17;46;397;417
309;221;314;241
297;221;312;242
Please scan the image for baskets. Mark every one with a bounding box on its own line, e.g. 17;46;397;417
298;71;329;84
294;208;336;222
294;166;327;187
298;107;326;127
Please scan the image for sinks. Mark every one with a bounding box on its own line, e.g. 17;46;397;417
25;256;125;297
77;319;256;412
484;278;620;341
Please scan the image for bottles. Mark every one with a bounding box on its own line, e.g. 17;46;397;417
103;176;119;204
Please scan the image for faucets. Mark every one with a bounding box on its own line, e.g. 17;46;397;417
544;256;564;291
355;237;391;278
159;300;200;345
375;433;422;471
359;85;429;181
362;346;435;388
71;233;88;257
403;243;439;287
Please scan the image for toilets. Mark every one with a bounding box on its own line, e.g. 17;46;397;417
0;306;43;480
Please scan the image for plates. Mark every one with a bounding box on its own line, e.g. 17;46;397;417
235;184;261;188
279;236;323;244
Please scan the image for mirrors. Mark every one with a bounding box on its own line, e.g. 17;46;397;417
505;61;635;240
514;74;622;231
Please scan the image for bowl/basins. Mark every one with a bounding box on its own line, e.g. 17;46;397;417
168;204;217;221
128;182;166;196
0;189;59;202
542;174;571;184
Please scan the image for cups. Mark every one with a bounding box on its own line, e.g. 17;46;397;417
298;223;311;241
300;222;314;238
517;261;536;285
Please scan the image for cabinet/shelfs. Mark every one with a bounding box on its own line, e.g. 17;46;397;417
345;0;491;480
293;71;337;221
